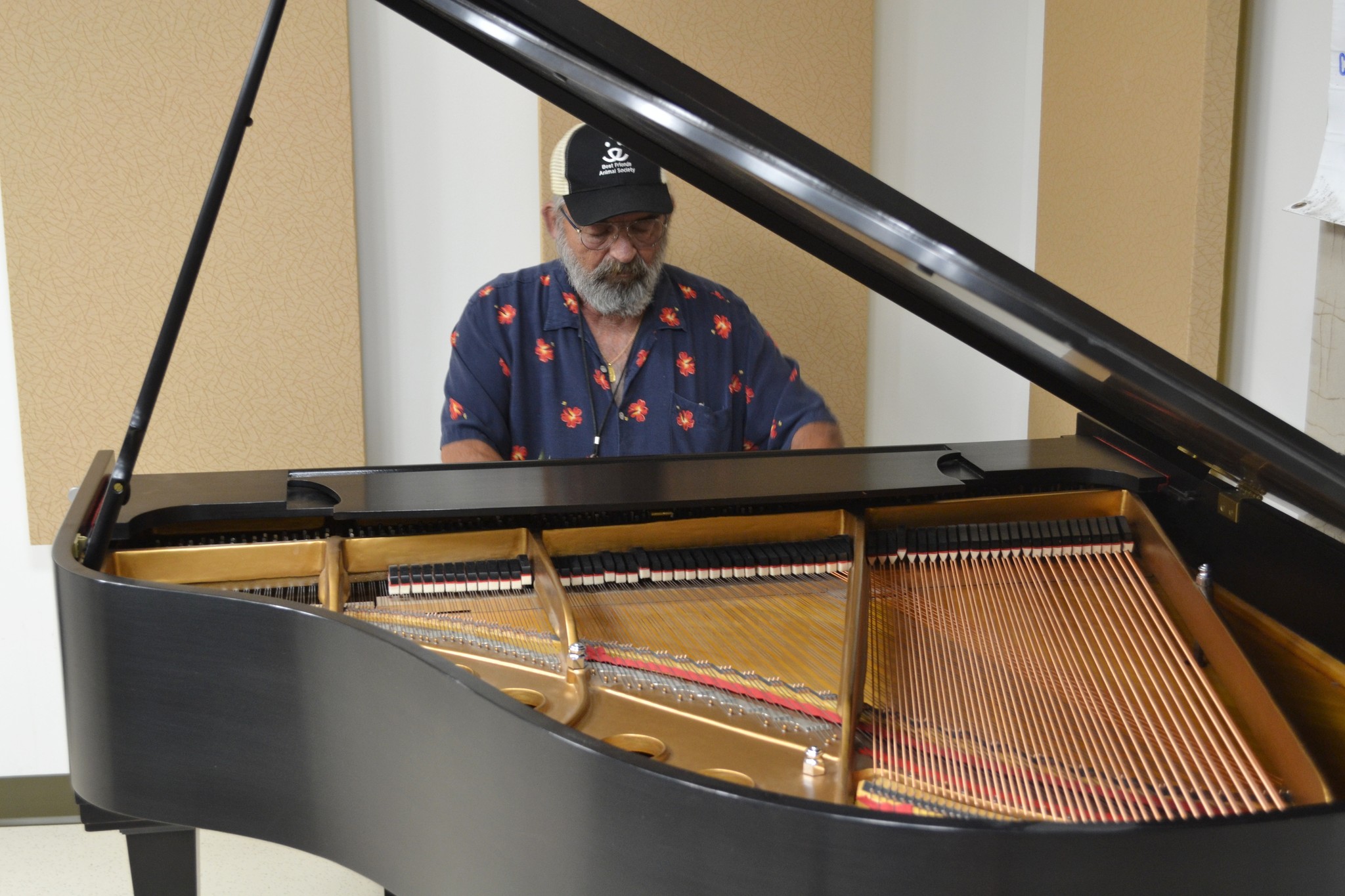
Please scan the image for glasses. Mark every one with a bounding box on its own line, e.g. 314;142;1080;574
560;207;669;251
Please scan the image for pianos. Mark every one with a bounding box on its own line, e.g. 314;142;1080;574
45;0;1345;896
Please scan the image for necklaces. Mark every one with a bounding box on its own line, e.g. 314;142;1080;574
565;290;647;460
605;321;640;383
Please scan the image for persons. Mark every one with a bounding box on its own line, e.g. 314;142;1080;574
438;119;846;464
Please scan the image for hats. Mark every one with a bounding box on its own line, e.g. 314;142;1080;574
551;122;674;225
